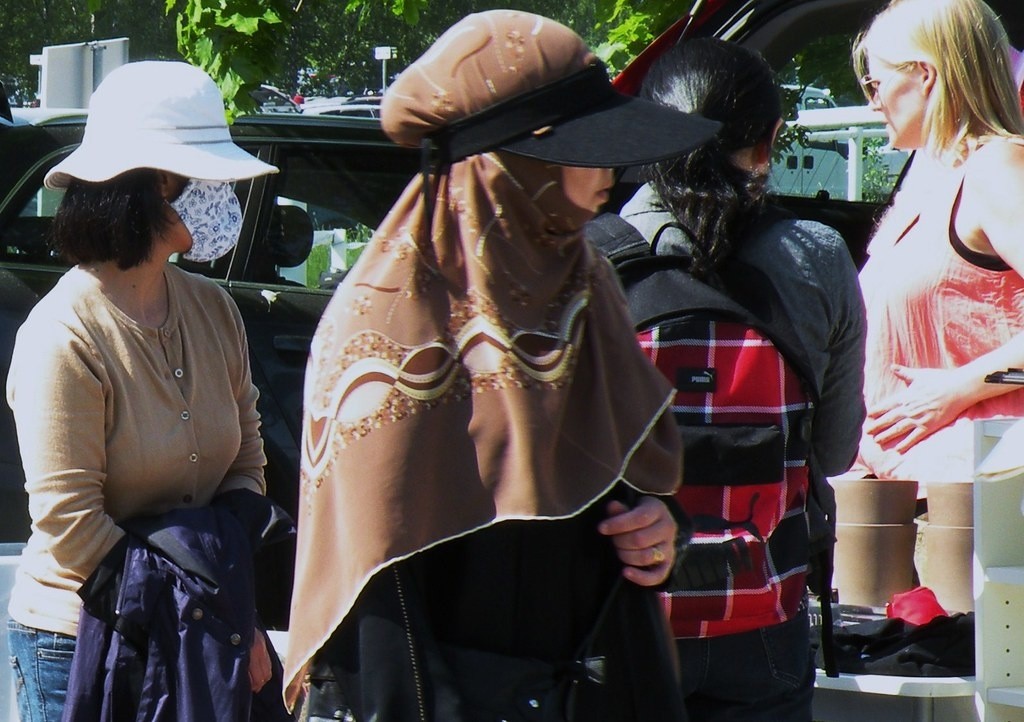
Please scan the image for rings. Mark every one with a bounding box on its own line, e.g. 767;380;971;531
652;547;666;563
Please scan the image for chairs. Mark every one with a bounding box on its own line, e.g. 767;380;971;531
274;206;316;286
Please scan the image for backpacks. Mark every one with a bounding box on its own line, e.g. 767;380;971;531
582;203;841;708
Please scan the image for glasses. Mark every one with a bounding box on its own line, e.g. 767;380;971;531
859;62;909;102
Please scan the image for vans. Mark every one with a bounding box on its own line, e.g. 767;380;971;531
761;84;853;199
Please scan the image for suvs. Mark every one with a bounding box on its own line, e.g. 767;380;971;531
0;82;434;542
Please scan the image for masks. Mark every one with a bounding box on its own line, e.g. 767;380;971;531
162;177;244;264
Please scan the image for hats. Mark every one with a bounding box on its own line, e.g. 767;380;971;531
41;58;280;193
414;58;726;177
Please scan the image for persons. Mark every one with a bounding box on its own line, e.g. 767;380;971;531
280;11;871;722
6;57;280;722
829;0;1024;581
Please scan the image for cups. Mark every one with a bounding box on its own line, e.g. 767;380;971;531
827;477;918;608
914;481;973;614
